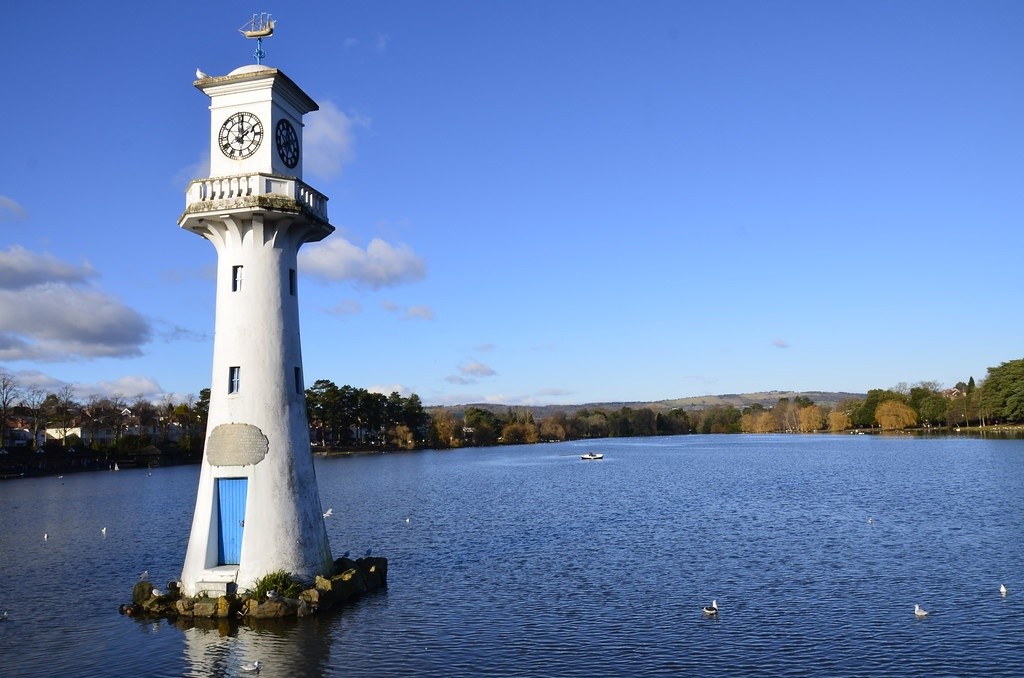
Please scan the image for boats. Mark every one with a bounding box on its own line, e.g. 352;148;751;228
579;453;604;460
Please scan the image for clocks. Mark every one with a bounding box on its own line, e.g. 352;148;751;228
218;112;264;161
275;119;300;169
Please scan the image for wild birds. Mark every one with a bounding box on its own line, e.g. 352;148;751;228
139;571;149;582
700;599;720;616
323;508;334;519
913;604;928;616
101;527;107;532
44;534;49;539
152;588;165;597
404;518;410;522
240;661;263;672
1000;584;1008;595
